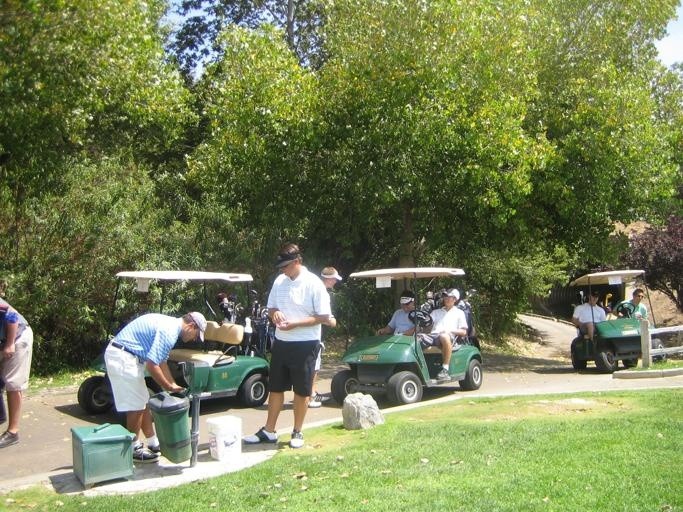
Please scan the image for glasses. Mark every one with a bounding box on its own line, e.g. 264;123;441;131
636;295;644;299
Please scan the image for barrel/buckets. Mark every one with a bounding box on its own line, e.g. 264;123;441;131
205;418;241;460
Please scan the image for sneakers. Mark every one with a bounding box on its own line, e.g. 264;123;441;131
289;431;304;448
244;427;278;444
0;430;19;448
436;369;450;380
308;393;331;407
132;444;160;463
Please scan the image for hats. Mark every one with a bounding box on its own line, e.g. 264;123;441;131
442;288;461;300
399;296;414;305
189;311;208;342
275;254;302;268
320;269;344;280
589;288;601;298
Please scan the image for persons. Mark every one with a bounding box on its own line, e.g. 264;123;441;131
307;265;344;409
416;288;468;381
377;290;416;336
572;289;606;341
239;242;332;448
613;289;647;320
104;309;208;463
0;297;35;447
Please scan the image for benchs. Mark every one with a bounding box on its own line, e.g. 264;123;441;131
408;319;461;354
169;320;244;367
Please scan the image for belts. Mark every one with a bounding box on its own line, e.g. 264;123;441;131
109;340;135;356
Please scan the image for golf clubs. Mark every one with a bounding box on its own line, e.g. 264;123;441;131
227;289;268;321
421;288;476;314
605;293;612;306
580;291;584;304
